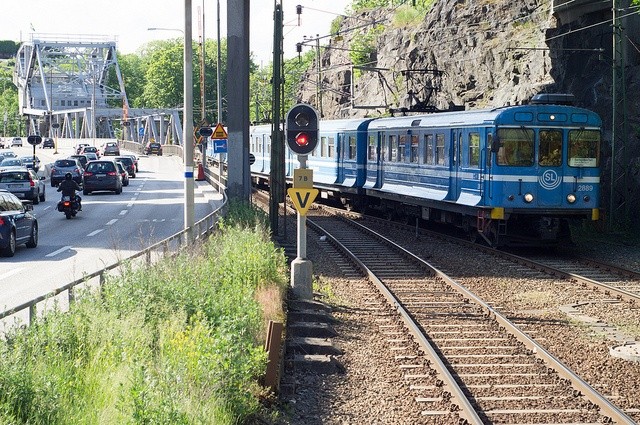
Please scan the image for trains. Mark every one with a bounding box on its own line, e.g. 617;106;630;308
206;94;602;258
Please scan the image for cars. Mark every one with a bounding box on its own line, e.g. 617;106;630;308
0;189;37;257
83;152;98;160
115;162;128;186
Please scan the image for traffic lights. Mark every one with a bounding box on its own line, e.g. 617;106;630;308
287;105;320;154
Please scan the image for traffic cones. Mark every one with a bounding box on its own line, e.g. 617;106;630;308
195;164;206;181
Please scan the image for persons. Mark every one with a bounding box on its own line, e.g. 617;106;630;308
56;173;82;211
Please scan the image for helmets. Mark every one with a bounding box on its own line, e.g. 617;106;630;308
65;173;72;180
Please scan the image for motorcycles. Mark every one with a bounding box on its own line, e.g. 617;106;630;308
55;183;84;219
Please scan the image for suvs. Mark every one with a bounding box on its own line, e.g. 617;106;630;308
42;138;55;148
50;159;83;186
73;144;90;154
1;158;26;166
144;141;162;156
122;155;140;172
115;156;136;178
11;138;22;146
0;165;46;204
81;147;101;159
71;155;88;169
81;161;122;195
101;142;120;156
